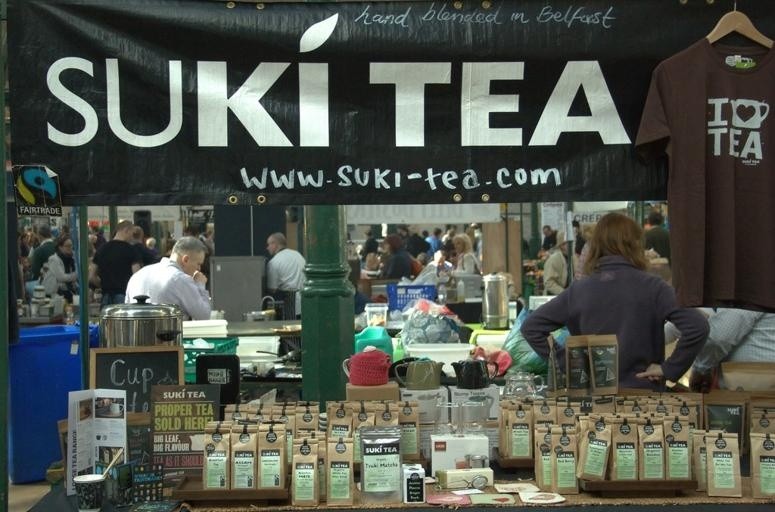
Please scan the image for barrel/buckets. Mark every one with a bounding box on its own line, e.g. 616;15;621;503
6;324;88;484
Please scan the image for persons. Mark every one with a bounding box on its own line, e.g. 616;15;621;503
166;222;215;293
359;223;482;280
265;233;306;320
21;220;163;311
687;306;774;390
518;210;712;391
642;210;669;258
542;220;595;296
124;236;211;321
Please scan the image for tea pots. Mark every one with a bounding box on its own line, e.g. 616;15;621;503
452;351;500;389
502;368;545;401
394;357;444;392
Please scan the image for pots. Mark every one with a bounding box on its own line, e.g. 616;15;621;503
97;293;183;347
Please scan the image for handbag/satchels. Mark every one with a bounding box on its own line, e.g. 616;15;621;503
462;253;480;274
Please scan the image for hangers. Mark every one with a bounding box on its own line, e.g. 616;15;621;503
654;0;775;78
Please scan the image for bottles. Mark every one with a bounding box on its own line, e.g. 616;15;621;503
457;279;467;304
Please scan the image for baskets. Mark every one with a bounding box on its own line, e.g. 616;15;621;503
387;282;438;311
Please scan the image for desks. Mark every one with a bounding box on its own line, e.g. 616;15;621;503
180;237;775;512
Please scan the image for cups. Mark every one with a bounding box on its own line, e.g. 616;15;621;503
464;453;488;467
73;473;105;512
435;401;485;436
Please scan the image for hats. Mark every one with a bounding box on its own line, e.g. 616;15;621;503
554;231;575;248
